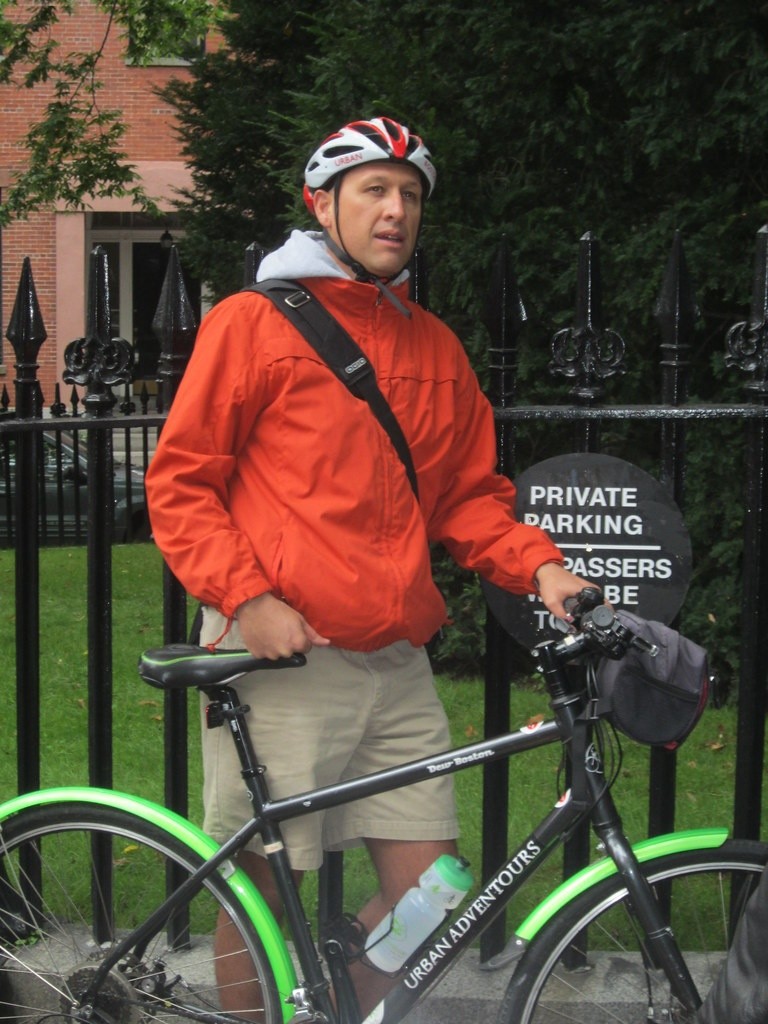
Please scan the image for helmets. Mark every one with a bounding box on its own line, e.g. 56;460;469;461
303;118;436;218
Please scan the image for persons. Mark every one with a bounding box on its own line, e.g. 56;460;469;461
145;117;614;1024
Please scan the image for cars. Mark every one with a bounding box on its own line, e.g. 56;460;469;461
0;429;153;550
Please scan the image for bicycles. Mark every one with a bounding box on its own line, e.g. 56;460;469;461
0;588;768;1024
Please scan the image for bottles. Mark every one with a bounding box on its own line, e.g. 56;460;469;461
364;853;473;973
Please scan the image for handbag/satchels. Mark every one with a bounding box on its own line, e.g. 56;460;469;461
597;609;711;751
